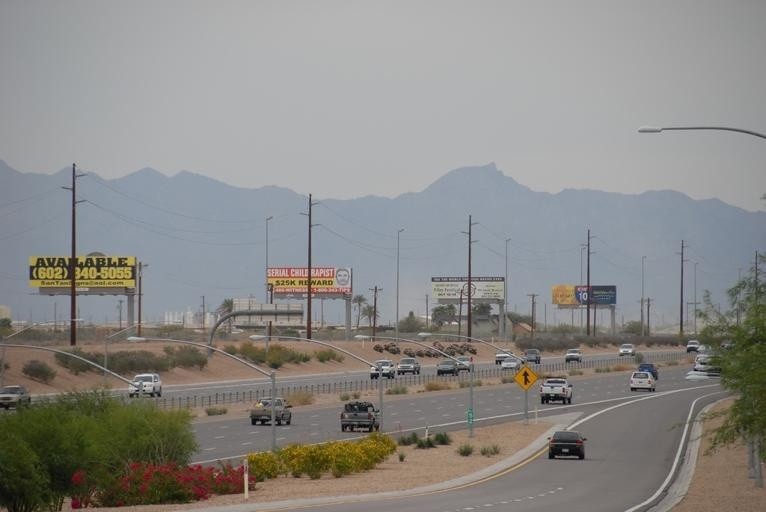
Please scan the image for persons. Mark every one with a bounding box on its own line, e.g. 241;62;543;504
335;269;351;285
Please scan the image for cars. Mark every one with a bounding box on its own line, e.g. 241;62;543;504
630;363;659;391
496;348;541;371
370;360;396;379
619;344;636;356
128;373;162;397
398;358;421;375
250;397;293;425
0;385;31;409
340;401;380;432
565;348;583;363
539;376;573;404
687;340;721;376
548;431;588;459
437;356;473;377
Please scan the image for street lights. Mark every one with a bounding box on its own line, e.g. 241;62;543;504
249;334;383;434
127;337;276;452
418;332;529;425
355;336;474;437
104;324;146;375
638;124;766;141
0;319;83;387
